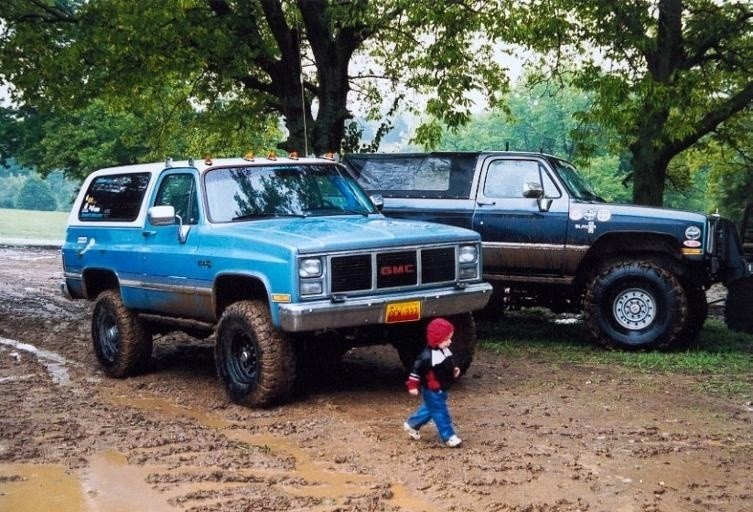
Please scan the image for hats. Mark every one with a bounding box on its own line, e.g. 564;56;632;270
426;317;455;348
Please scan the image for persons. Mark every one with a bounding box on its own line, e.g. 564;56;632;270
401;318;463;448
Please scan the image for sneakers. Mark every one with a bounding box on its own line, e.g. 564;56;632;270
404;422;420;440
445;434;462;447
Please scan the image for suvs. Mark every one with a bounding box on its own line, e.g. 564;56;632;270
59;150;492;411
327;149;752;352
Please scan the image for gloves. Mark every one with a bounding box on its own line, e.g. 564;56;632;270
406;373;421;395
